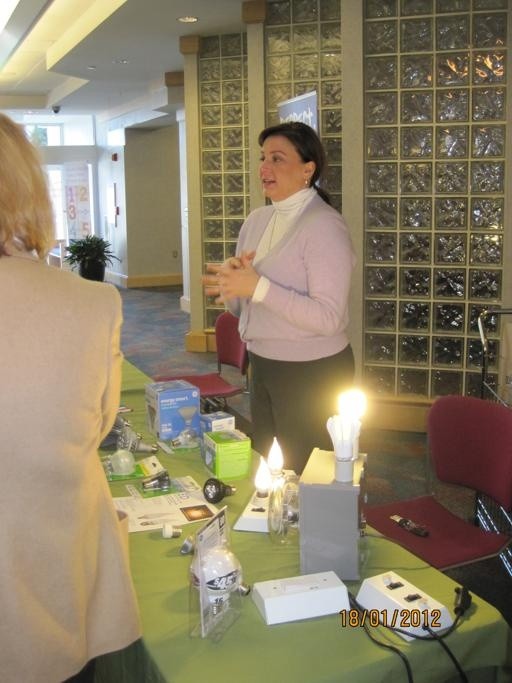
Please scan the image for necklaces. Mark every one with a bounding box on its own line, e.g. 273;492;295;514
268;196;310;254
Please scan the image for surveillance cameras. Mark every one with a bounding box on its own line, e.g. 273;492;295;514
52;106;60;113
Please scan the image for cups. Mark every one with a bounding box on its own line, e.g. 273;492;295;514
268;492;300;545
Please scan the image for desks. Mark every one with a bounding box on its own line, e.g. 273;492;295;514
97;359;505;683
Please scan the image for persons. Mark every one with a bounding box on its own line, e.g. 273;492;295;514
0;113;169;681
201;120;356;484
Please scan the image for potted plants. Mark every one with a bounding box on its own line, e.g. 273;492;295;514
63;234;124;281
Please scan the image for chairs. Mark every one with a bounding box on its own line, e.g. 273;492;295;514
153;306;250;416
362;395;512;574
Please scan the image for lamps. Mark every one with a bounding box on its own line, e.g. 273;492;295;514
253;434;286;507
326;386;365;481
